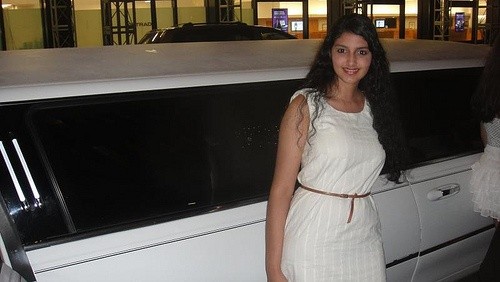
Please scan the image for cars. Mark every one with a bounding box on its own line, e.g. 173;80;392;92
0;38;497;282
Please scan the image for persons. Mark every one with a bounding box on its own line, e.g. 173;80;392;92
469;36;500;281
262;13;403;282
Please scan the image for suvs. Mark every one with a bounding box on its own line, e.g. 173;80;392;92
138;20;299;44
